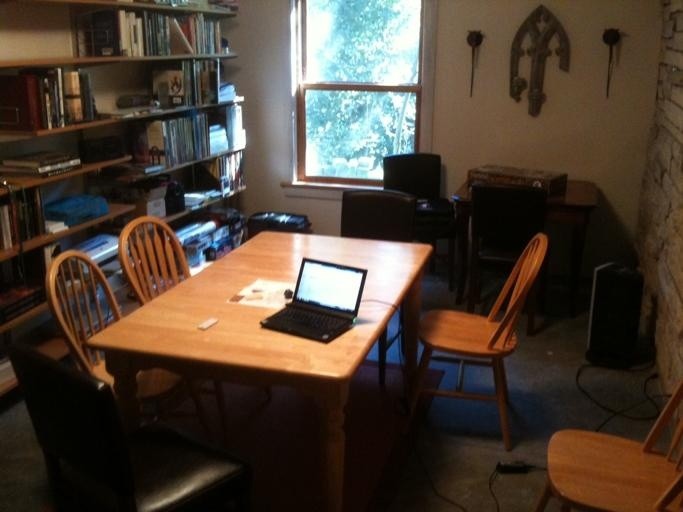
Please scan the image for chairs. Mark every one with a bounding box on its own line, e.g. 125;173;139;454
533;375;682;512
117;216;269;411
39;250;212;462
406;233;550;451
462;182;551;336
1;336;245;512
380;148;457;293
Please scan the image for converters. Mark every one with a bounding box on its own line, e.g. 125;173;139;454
496;459;527;473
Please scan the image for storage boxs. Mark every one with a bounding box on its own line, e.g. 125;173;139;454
466;165;568;197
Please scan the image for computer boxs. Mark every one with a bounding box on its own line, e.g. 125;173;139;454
247;211;313;239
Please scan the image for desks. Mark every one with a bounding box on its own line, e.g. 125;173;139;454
79;224;436;510
445;176;596;304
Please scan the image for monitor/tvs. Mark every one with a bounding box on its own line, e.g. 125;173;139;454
383;153;441;201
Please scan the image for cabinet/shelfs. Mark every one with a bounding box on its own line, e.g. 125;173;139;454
0;2;143;400
127;3;248;219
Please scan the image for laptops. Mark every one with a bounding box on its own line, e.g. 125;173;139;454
259;256;367;343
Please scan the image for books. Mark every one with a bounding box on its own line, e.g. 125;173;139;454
1;278;46;323
88;8;221;57
2;152;82;177
2;187;109;249
1;68;95;131
191;153;244;197
122;104;245;174
148;58;219;107
